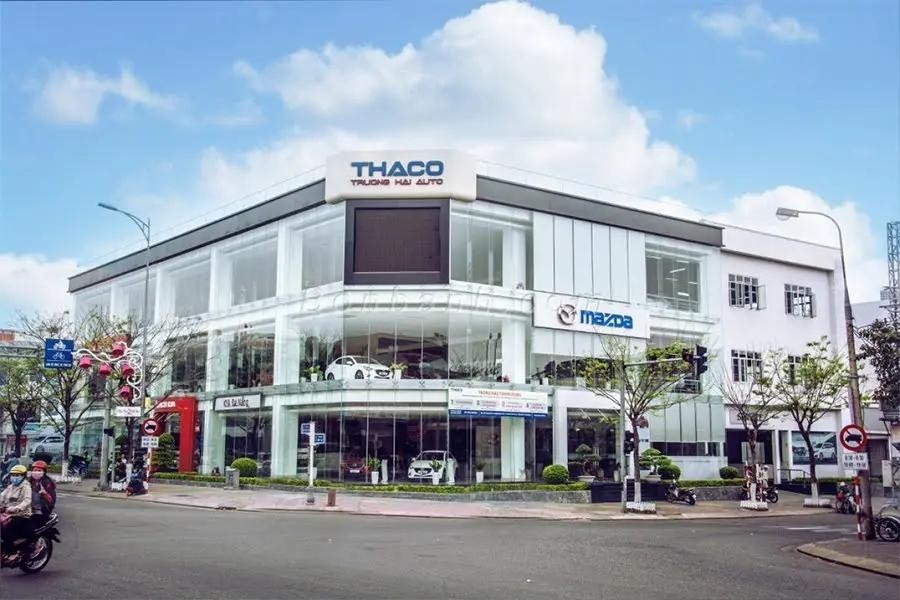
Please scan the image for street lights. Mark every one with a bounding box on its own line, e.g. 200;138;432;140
97;201;152;419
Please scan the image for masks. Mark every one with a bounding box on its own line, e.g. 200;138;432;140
31;472;45;479
10;476;25;485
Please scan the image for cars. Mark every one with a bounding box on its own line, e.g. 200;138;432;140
324;355;392;379
29;434;64;456
812;442;836;461
408;450;460;481
297;442;349;476
336;446;373;477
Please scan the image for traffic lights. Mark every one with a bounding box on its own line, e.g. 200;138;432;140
692;344;707;380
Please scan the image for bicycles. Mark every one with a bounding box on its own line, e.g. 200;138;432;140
834;485;857;515
775;207;878;540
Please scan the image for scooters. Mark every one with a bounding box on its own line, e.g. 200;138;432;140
741;482;778;503
0;512;61;574
664;483;697;505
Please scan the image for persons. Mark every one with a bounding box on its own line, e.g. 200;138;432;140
121;453;127;464
0;464;33;566
2;451;19;488
79;450;91;474
106;458;126;482
841;478;862;513
745;465;768;500
20;460;57;560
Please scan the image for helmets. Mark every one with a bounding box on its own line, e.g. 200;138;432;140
10;464;27;478
32;460;48;474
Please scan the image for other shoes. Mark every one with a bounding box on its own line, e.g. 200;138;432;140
1;554;21;568
30;544;45;559
